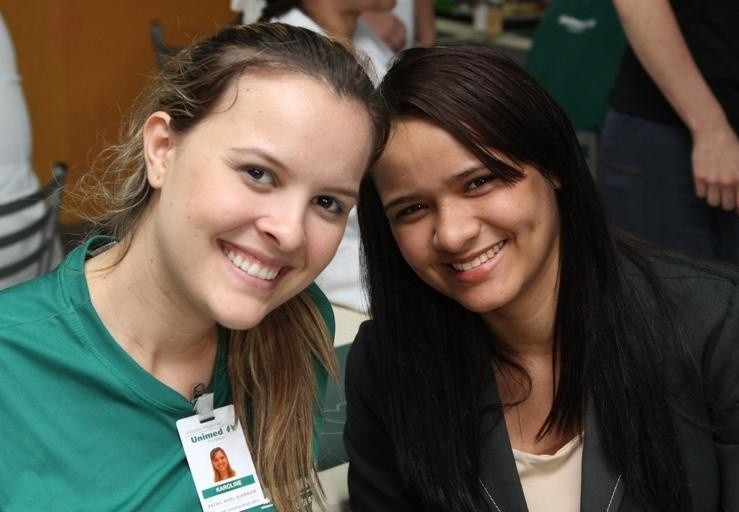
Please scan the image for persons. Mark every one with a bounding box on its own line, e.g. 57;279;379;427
234;0;406;470
209;446;236;482
589;1;738;279
343;37;739;508
1;16;393;512
360;1;438;57
0;15;69;293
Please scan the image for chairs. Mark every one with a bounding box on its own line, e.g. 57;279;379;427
0;159;69;293
150;16;185;75
